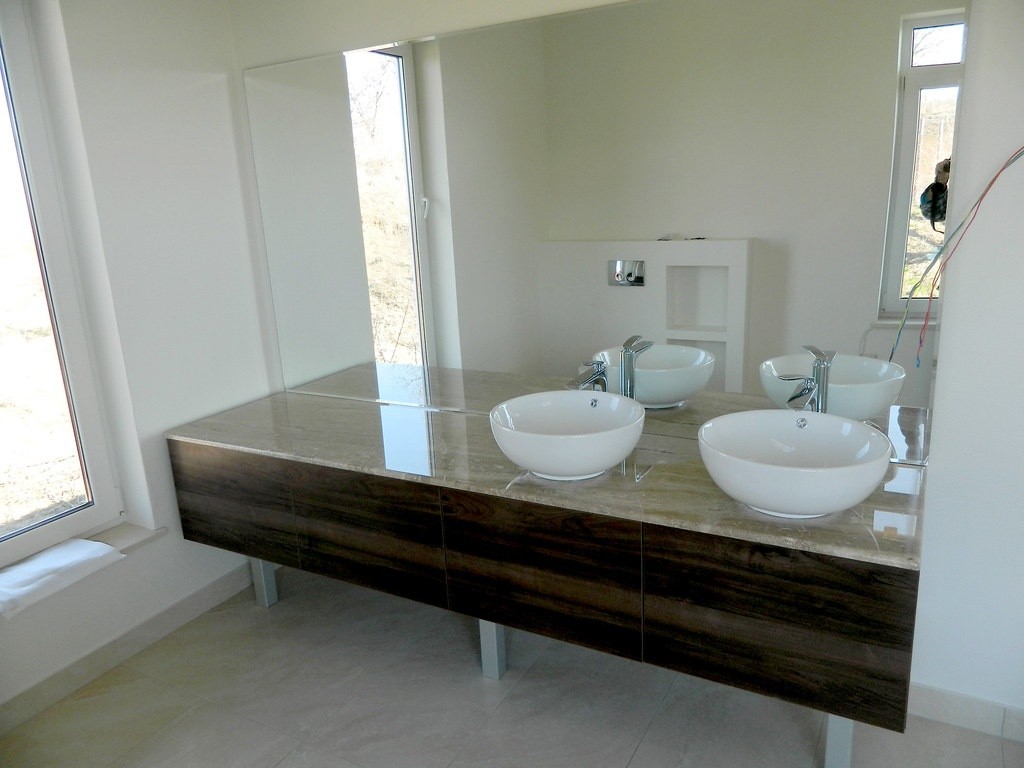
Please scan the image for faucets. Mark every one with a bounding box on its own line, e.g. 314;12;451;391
562;358;609;394
802;343;839;414
777;373;820;412
618;335;656;399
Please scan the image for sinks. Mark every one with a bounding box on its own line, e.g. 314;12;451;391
758;351;906;421
695;406;893;520
589;342;717;410
488;389;647;483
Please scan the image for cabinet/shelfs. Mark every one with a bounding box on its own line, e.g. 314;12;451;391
167;439;919;734
540;237;785;394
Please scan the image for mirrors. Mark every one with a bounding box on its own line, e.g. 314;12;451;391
243;1;969;468
